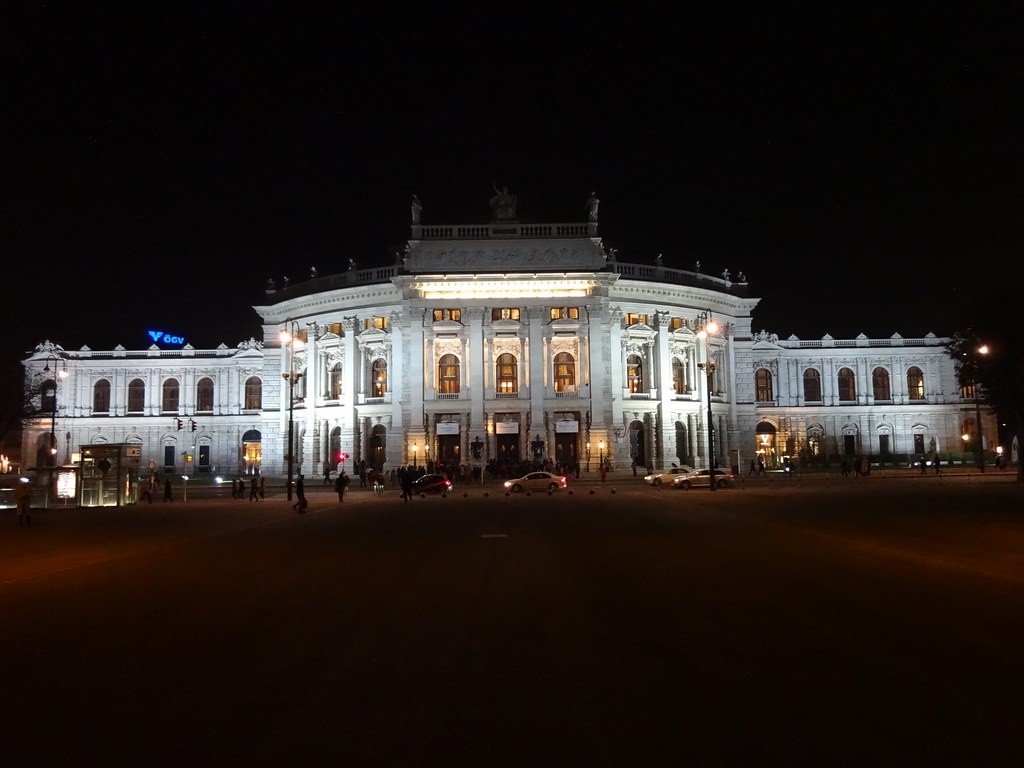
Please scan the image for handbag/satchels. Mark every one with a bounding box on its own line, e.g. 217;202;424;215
299;496;308;509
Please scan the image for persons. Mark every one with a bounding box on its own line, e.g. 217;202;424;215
292;475;304;514
411;194;422;224
140;479;151;505
599;456;611;482
391;464;434;485
933;454;940;474
646;461;654;475
748;459;766;476
920;456;928;475
447;462;481;483
164;479;173;503
494;184;512;219
323;468;333;485
589;191;600;222
16;482;33;527
487;459;580;479
995;454;1008;472
250;475;265;501
840;455;850;477
400;467;412;503
335;474;346;504
359;461;374;488
631;458;636;476
232;476;245;500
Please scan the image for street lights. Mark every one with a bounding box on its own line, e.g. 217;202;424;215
599;439;604;466
699;308;718;491
281;317;303;500
963;333;988;472
412;442;417;468
44;354;66;489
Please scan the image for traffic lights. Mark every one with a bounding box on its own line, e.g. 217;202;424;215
178;419;183;430
192;421;197;432
340;453;344;461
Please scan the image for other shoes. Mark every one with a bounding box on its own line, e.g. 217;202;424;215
292;505;296;510
298;510;306;514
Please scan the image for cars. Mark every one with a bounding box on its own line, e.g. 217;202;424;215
644;466;691;485
504;471;567;492
410;474;453;494
672;469;734;488
